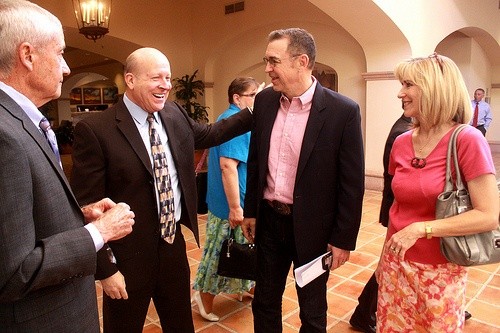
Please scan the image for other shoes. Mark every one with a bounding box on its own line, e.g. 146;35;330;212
465;311;471;320
349;305;377;333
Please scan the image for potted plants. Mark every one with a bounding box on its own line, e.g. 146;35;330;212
172;68;210;167
55;120;75;154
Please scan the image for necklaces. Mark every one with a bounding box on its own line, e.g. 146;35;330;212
416;139;429;153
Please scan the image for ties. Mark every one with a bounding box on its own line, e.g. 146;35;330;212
39;118;63;172
473;102;478;128
147;114;176;244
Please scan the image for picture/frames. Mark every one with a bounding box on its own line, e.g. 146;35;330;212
70;88;83;105
102;87;118;104
82;88;101;104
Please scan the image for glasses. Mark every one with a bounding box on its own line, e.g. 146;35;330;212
238;92;256;99
428;52;444;75
263;54;303;66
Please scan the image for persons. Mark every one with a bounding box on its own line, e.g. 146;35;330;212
191;77;258;321
468;88;492;137
0;0;136;333
240;27;366;333
374;55;499;333
350;102;472;333
69;47;274;333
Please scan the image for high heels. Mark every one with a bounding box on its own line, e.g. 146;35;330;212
239;290;255;302
191;290;219;321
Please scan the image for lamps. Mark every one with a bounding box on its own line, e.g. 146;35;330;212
71;0;112;44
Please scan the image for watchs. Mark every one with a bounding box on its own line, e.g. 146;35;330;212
425;220;433;239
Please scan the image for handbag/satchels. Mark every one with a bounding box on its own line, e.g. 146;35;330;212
437;124;500;266
217;229;260;281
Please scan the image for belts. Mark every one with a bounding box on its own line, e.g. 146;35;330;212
266;200;293;215
